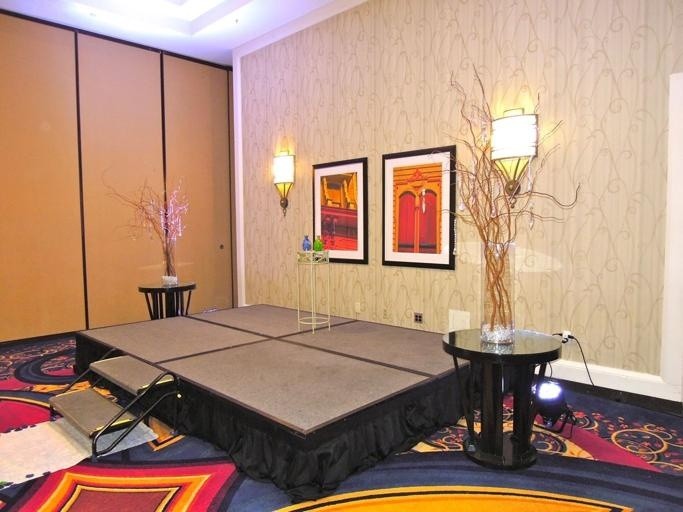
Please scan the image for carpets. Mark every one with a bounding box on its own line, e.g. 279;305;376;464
0;416;159;488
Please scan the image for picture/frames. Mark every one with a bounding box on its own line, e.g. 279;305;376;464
310;144;456;268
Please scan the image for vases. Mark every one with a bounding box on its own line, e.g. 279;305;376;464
302;234;322;251
161;235;180;286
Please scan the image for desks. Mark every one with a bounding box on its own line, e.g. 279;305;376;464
440;326;562;470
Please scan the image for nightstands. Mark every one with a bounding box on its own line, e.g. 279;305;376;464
136;280;195;321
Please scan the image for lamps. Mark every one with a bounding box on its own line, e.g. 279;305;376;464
530;379;577;432
489;106;540;209
270;150;294;218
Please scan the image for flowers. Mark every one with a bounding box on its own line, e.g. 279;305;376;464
112;179;191;277
